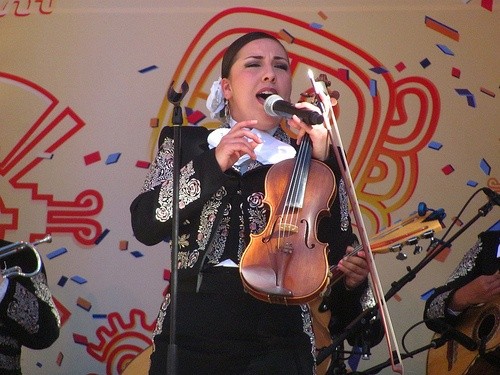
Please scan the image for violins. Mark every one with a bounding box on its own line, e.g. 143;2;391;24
239;73;337;304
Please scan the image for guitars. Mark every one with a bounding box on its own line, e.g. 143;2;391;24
121;202;446;375
425;296;500;375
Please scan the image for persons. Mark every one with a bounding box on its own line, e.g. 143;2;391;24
0;240;61;375
422;230;500;332
130;31;383;375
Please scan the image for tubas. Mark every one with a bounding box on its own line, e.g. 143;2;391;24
0;233;53;277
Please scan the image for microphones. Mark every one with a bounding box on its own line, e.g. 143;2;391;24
482;186;500;207
263;94;324;125
428;318;477;351
